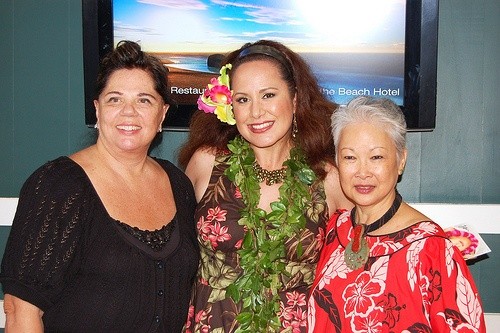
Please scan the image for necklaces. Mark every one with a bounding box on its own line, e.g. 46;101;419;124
251;161;288;187
343;187;402;271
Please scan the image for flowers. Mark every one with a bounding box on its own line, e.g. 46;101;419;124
197;64;238;126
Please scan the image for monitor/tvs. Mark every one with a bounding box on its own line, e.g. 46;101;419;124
80;0;439;134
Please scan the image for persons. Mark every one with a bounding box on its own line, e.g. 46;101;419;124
305;93;487;333
0;39;201;333
178;39;357;333
